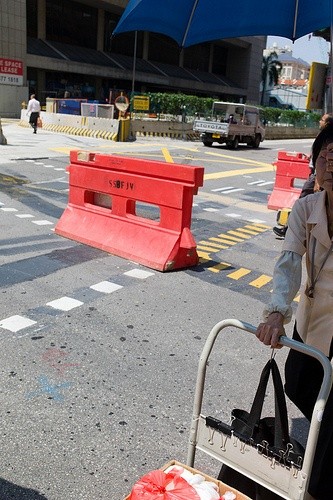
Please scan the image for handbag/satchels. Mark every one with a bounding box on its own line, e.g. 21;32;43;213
217;359;305;500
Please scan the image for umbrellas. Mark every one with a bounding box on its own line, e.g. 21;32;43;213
110;0;333;50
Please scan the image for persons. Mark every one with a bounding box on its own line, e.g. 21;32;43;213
256;123;333;500
273;112;333;238
26;94;41;134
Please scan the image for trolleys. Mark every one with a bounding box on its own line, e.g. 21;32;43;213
123;318;333;500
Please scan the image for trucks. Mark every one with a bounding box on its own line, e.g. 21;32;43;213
192;101;269;150
